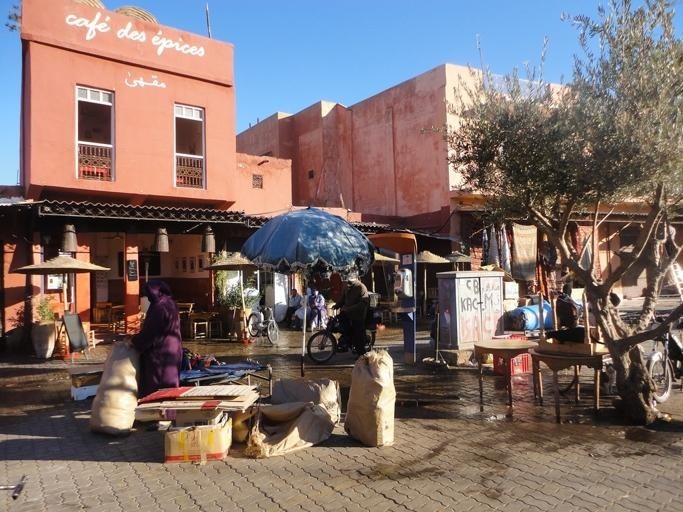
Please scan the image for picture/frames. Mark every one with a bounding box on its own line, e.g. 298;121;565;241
400;252;414;266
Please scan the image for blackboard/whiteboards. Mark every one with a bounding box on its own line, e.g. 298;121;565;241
63;314;88;351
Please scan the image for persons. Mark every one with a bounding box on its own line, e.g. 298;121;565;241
285;274;370;355
132;278;182;432
556;284;579;327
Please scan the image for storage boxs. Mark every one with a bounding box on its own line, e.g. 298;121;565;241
161;417;233;464
168;412;229;431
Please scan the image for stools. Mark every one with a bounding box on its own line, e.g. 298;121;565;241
208;317;223;338
190;319;208;340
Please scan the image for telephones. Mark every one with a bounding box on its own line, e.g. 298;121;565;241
394;269;412;298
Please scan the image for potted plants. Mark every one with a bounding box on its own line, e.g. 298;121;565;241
226;281;260;342
32;296;57;359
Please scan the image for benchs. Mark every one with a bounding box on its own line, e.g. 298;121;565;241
108;314;138;335
176;303;195;318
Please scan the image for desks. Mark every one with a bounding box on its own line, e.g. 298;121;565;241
472;291;612;425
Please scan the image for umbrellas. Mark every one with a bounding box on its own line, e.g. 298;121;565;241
12;251;112;355
240;204;375;378
374;251;469;320
203;252;260;342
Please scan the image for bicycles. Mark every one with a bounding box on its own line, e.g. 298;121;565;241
306;306;376;362
644;313;683;404
245;301;283;344
